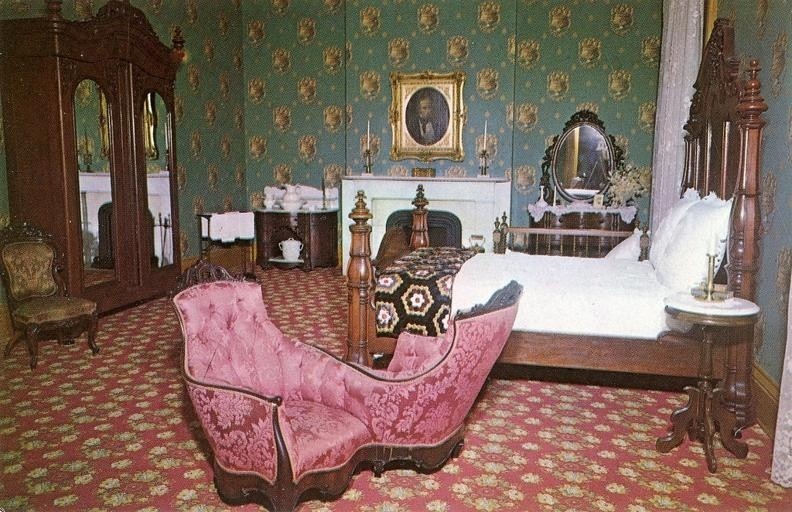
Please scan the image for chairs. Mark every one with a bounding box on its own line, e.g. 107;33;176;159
2;221;100;371
166;258;522;511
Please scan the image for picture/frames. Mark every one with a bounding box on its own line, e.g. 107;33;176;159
388;70;465;162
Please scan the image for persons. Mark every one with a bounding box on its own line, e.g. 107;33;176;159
405;95;447;149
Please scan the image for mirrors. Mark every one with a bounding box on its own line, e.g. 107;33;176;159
537;110;627;205
143;87;174;271
73;76;118;292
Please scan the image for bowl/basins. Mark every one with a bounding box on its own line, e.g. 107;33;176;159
277;199;305;209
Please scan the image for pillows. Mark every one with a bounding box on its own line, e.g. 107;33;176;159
649;188;734;294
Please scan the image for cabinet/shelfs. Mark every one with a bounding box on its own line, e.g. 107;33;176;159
527;205;639;258
255;207;340;271
1;2;185;317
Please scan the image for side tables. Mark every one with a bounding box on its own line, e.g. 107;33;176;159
655;296;760;472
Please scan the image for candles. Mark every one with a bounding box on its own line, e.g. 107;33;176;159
367;120;370;151
484;121;487;150
707;216;716;255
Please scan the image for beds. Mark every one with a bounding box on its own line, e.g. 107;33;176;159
342;18;769;428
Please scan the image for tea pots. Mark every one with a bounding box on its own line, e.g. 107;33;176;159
282;182;303;202
